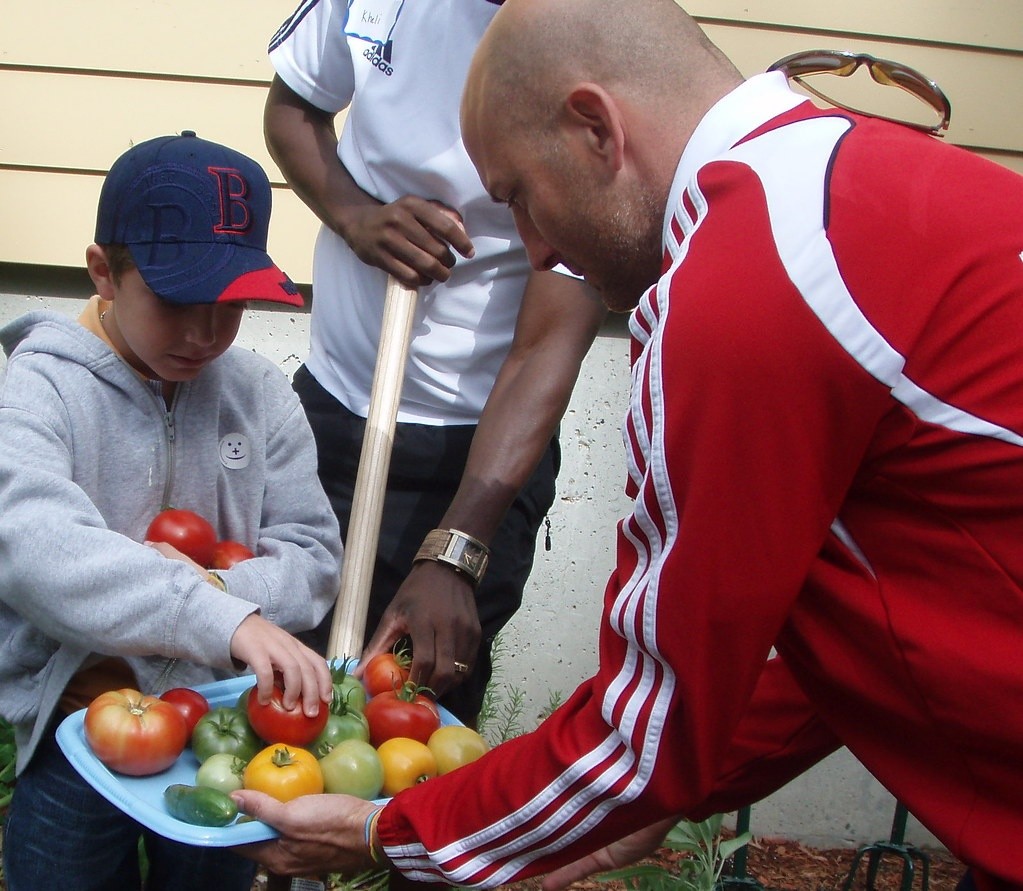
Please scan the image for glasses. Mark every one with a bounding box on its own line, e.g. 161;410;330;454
766;50;952;138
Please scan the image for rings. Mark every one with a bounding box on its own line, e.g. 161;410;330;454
455;662;468;673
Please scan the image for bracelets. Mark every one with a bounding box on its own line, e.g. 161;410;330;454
364;805;394;868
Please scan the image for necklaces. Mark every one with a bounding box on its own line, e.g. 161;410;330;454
100;310;107;322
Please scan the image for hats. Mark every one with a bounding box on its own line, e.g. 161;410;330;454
94;131;304;307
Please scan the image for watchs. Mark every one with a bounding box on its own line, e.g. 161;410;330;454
412;528;491;587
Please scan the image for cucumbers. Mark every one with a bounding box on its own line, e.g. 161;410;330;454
163;783;238;826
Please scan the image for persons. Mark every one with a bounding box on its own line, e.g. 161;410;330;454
228;0;1023;891
0;130;343;891
262;0;608;732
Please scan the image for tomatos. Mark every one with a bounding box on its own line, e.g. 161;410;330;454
146;509;216;569
207;540;257;570
85;652;492;803
